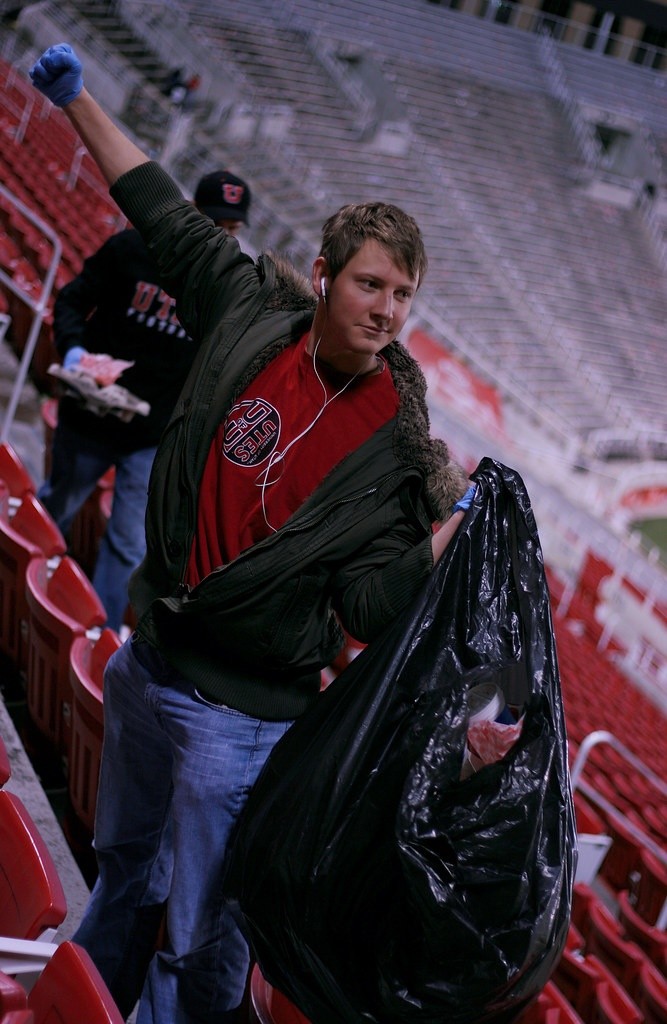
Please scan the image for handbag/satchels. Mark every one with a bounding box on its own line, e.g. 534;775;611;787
222;459;577;1024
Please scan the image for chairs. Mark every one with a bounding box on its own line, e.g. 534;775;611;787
0;61;667;1024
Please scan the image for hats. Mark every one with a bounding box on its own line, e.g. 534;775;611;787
195;171;251;228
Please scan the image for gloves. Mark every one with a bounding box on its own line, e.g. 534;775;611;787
29;42;83;107
453;483;479;513
63;345;87;367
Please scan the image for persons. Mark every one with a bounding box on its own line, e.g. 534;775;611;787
29;44;478;1024
119;68;200;164
34;171;252;641
